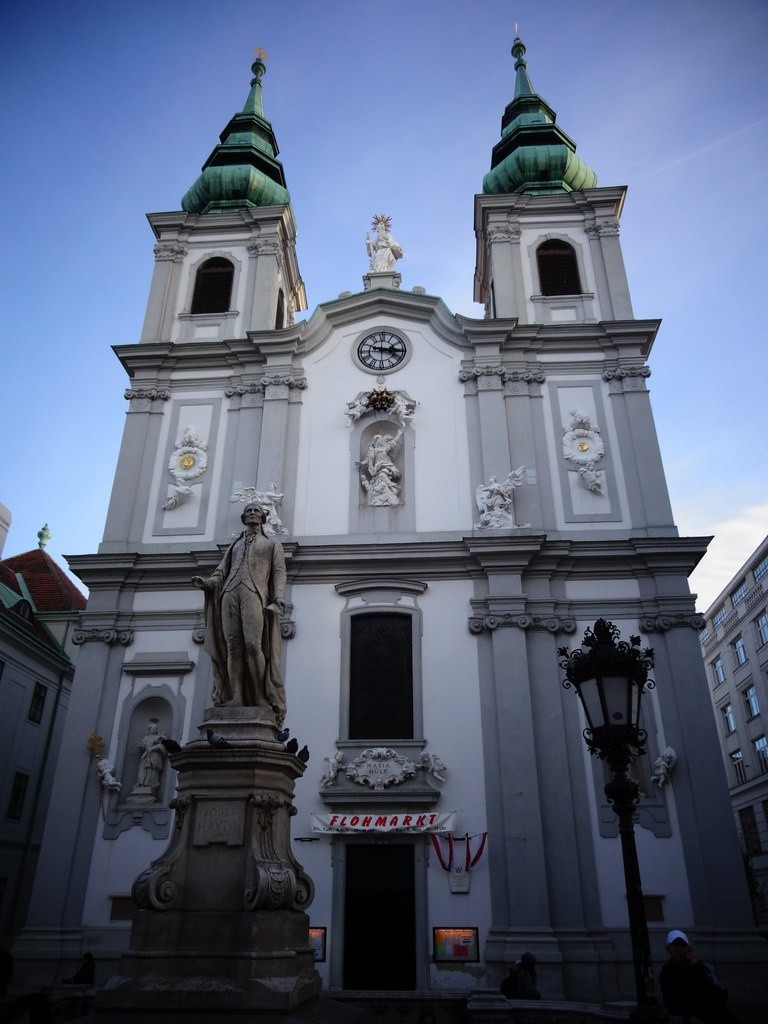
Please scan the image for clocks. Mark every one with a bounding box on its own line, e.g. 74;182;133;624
350;326;414;375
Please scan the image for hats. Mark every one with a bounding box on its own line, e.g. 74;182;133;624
667;930;689;946
516;959;521;964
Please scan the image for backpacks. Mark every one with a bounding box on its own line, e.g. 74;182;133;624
500;967;525;998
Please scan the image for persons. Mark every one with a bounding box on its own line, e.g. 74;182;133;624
96;762;122;793
386;395;414;427
61;952;96;985
352;428;414;494
585;462;602;489
345;395;369;428
657;929;731;1024
480;476;509;513
191;501;290;729
160;477;195;511
262;481;285;536
321;751;349;787
133;722;169;788
365;220;401;272
649;749;674;789
413;751;446;784
498;952;543;1001
